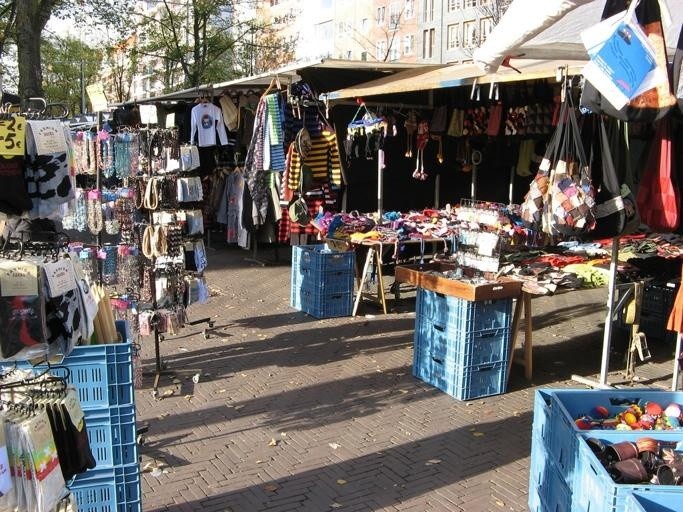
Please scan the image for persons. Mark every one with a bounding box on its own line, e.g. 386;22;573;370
201;114;213;129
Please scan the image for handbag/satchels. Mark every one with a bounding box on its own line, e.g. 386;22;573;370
521;158;680;239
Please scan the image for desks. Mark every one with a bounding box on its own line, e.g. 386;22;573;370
325;236;451;317
507;275;659;380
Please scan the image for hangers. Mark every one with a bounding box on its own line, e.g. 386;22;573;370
191;87;264;107
213;161;246;177
